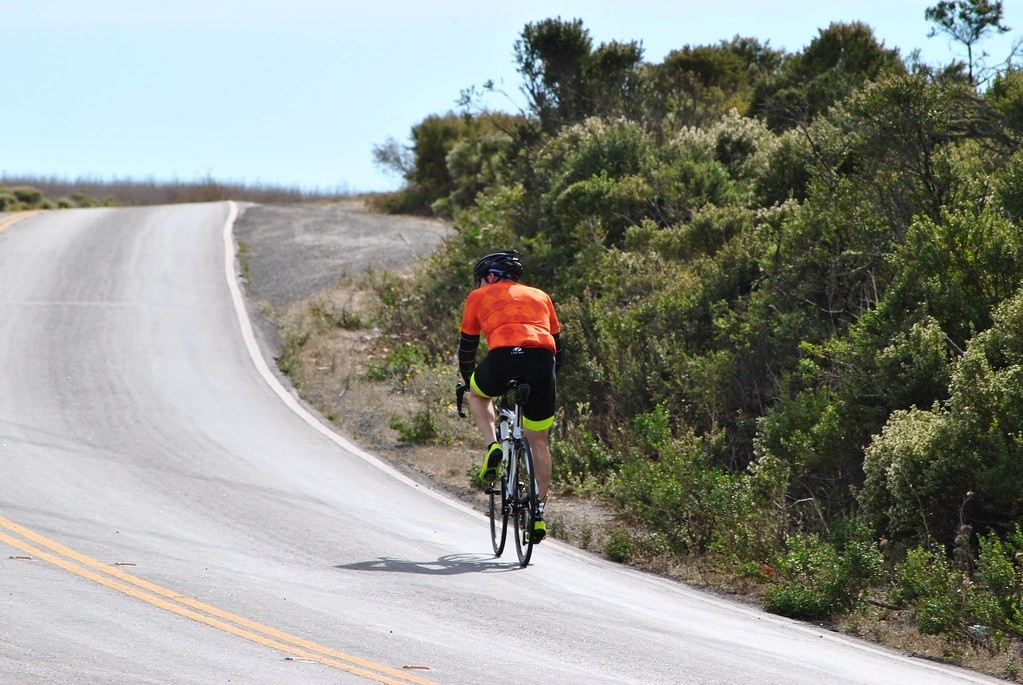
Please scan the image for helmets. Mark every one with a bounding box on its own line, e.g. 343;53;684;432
473;250;523;280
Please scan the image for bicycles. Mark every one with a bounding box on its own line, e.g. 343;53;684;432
455;379;537;568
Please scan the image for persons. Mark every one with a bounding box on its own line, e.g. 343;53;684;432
458;250;563;544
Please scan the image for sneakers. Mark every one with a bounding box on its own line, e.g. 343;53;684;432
479;443;503;483
525;517;546;540
